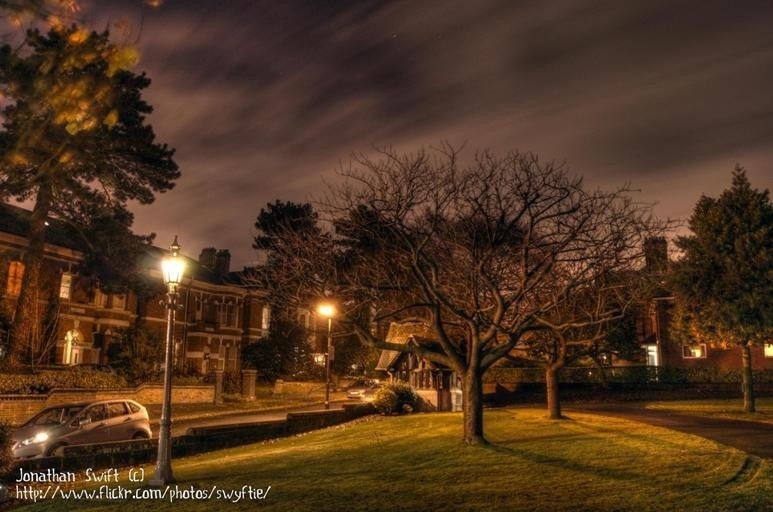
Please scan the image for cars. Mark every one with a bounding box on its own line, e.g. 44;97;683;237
346;377;383;399
69;364;118;375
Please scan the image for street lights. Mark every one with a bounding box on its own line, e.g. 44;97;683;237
318;301;335;408
147;235;188;486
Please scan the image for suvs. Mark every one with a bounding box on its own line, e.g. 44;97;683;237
5;399;152;463
